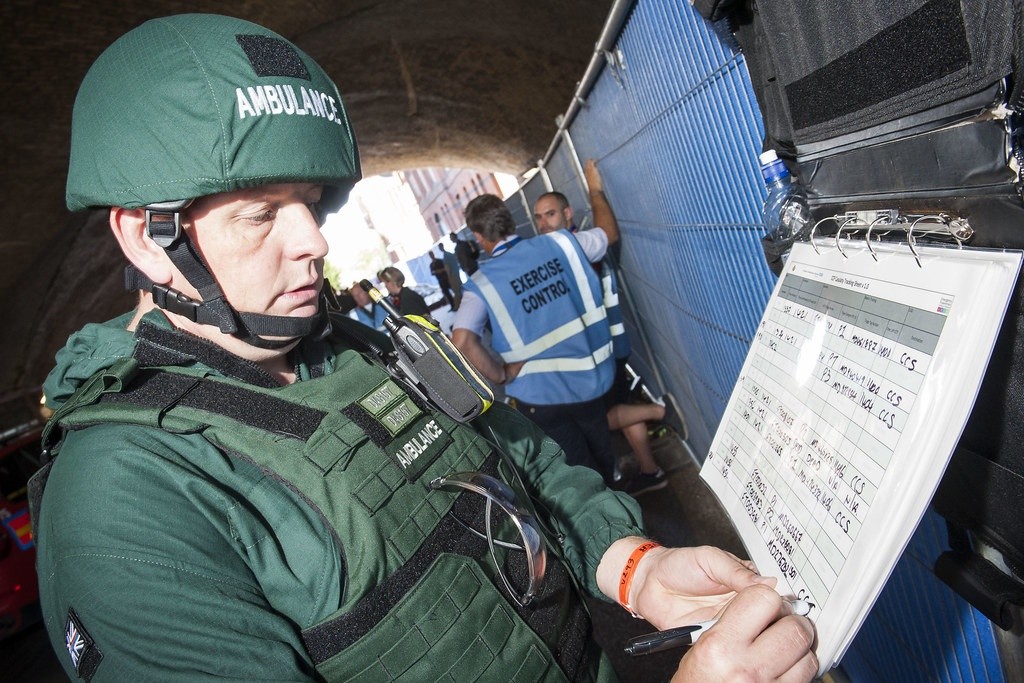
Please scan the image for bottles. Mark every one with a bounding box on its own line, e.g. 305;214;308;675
759;149;818;268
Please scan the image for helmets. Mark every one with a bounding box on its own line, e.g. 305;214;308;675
65;13;362;213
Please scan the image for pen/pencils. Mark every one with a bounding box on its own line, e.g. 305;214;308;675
620;597;814;657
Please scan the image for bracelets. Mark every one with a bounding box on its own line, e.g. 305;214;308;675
617;541;665;618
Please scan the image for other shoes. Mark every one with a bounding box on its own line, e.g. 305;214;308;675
626;467;668;497
658;392;688;441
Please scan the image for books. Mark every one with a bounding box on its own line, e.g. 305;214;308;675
695;229;1022;683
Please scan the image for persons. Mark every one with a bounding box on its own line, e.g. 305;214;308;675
36;23;822;683
448;155;621;496
348;231;479;342
526;189;687;499
